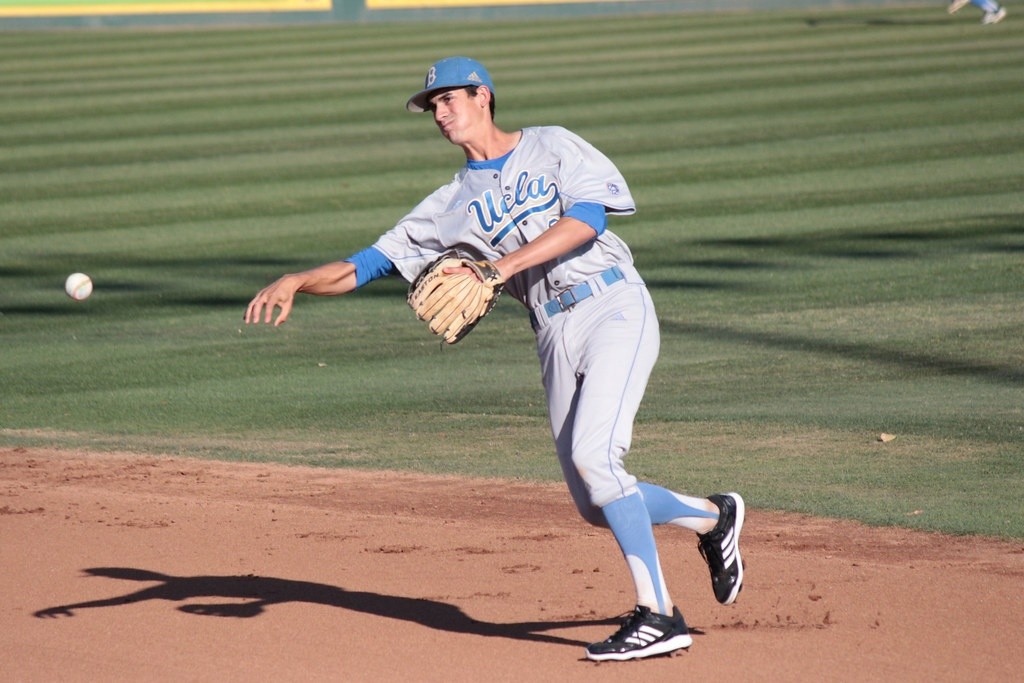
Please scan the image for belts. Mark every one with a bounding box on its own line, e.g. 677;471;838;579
529;266;623;328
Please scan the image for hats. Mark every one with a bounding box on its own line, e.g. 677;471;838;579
406;56;495;112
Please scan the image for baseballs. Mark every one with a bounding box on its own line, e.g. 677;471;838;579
65;270;95;301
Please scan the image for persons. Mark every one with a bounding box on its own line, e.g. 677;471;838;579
242;56;745;662
946;0;1008;25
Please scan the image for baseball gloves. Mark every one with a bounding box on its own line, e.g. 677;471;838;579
406;241;505;346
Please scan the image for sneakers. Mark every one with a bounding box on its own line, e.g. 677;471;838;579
585;605;693;664
698;493;745;606
